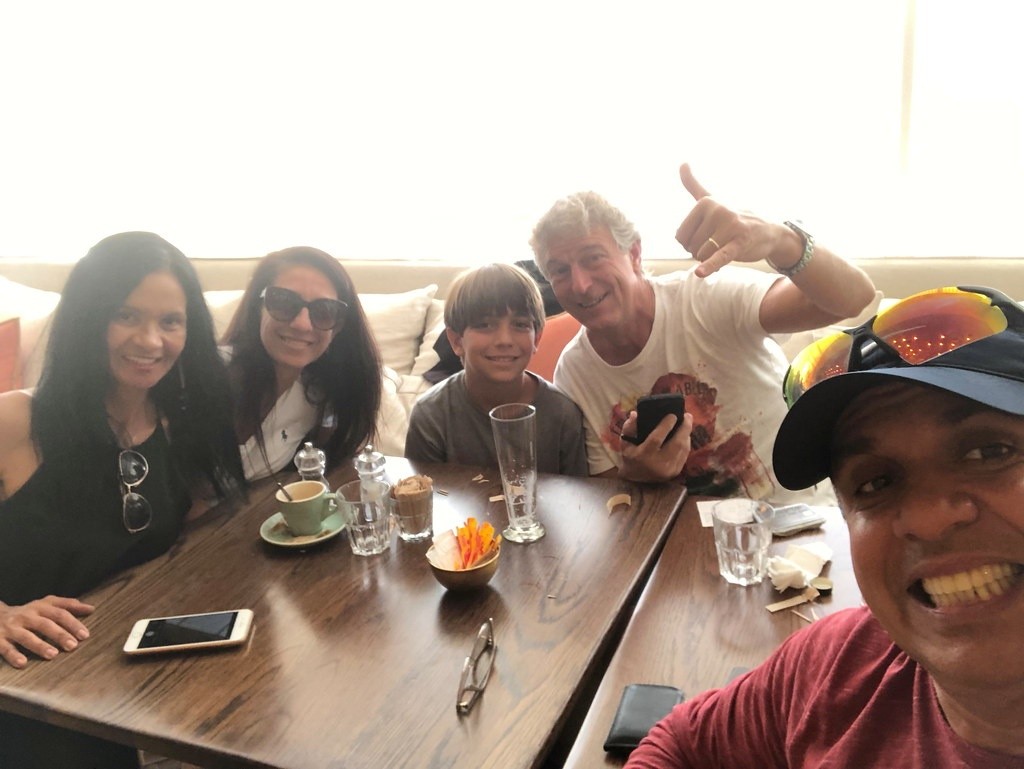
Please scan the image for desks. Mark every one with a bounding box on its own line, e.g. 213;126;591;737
0;450;864;769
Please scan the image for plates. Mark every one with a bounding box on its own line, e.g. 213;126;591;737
259;499;351;546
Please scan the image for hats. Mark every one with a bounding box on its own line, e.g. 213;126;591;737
771;321;1024;491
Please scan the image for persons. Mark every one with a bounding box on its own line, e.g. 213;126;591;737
530;163;877;505
403;263;589;478
0;231;248;666
626;288;1024;769
216;245;382;482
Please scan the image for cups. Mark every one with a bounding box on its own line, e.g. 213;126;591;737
710;497;776;586
335;479;391;557
275;481;342;537
489;403;547;545
385;484;435;544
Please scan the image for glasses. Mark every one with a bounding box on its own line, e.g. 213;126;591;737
259;286;351;331
117;449;153;533
780;286;1024;409
455;617;499;715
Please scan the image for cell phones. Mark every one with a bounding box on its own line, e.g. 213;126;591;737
635;393;687;446
122;609;254;656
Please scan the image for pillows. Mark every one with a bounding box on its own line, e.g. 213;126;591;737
357;283;449;374
0;275;63;393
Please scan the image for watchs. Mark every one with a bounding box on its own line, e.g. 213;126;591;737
765;220;815;277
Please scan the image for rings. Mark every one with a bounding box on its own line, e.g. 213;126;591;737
708;237;719;250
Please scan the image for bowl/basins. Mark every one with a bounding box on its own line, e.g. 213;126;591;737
426;536;500;590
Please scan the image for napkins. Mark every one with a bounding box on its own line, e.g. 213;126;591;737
696;500;725;527
763;544;833;591
